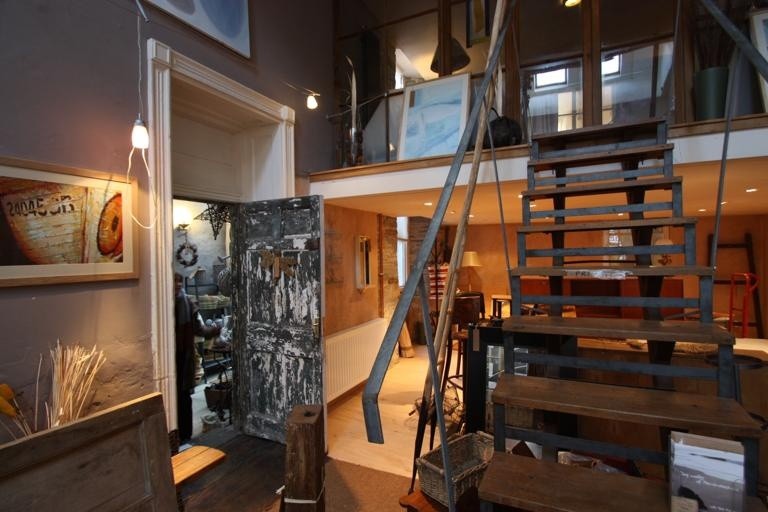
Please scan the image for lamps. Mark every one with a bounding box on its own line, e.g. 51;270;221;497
131;14;150;149
176;212;190;238
461;251;483;292
306;91;318;110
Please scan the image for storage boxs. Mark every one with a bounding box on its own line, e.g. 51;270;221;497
669;431;744;512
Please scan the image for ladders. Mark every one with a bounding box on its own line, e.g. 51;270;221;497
706;232;764;338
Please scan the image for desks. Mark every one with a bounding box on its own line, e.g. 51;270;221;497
399;489;472;512
492;295;512;320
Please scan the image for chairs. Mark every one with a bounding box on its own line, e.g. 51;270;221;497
441;296;480;398
457;292;484;374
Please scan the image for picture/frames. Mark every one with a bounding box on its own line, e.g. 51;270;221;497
0;155;140;289
397;72;470;158
603;224;671;267
749;8;768;112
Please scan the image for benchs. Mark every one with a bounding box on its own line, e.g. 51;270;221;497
169;444;225;486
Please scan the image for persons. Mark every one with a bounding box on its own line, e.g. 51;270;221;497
174;272;226;446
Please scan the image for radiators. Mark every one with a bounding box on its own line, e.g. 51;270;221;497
324;318;390;403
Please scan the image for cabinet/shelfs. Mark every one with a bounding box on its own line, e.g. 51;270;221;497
186;276;231;370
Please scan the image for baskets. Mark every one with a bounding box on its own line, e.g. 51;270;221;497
416;433;494;507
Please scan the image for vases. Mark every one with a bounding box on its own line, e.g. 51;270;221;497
693;65;726;120
